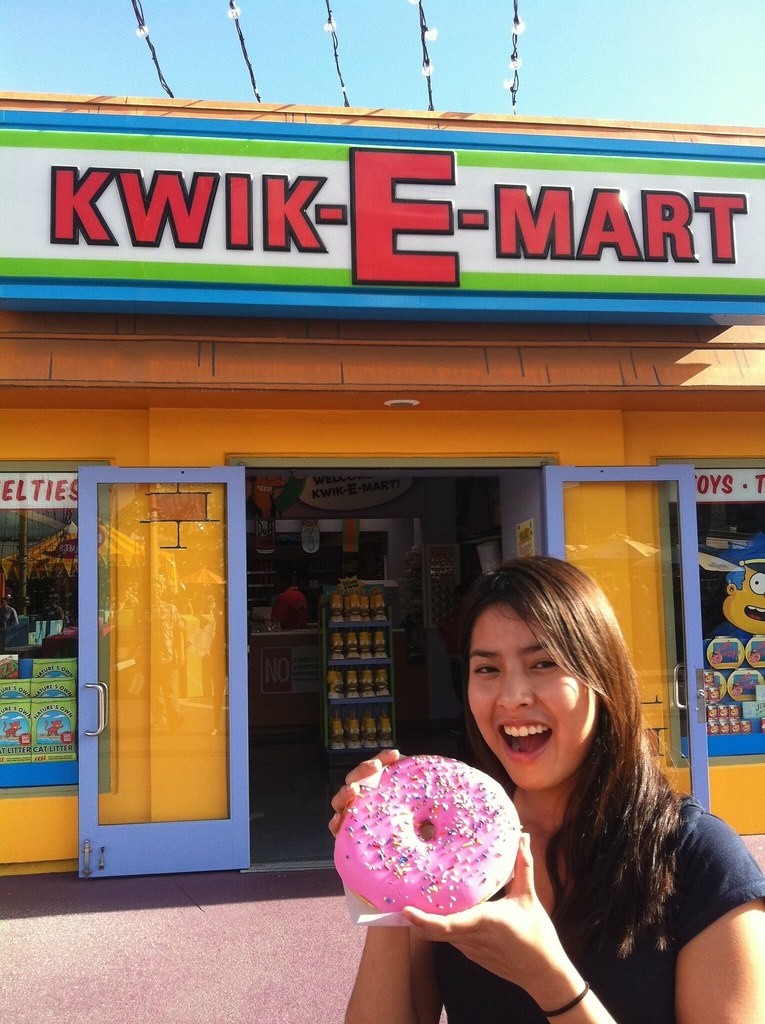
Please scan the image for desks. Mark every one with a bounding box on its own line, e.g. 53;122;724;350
41;634;77;658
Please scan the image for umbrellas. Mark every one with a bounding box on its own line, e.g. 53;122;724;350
181;569;226;584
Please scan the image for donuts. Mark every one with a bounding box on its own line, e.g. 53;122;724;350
335;755;521;915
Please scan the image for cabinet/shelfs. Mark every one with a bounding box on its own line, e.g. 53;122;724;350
247;551;388;626
318;584;399;753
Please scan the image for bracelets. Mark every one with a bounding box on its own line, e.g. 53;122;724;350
532;981;590;1016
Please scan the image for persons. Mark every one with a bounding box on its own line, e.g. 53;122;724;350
135;582;184;732
329;555;765;1024
0;598;19;626
210;615;225;735
439;584;473;700
115;583;139;609
270;569;308;630
